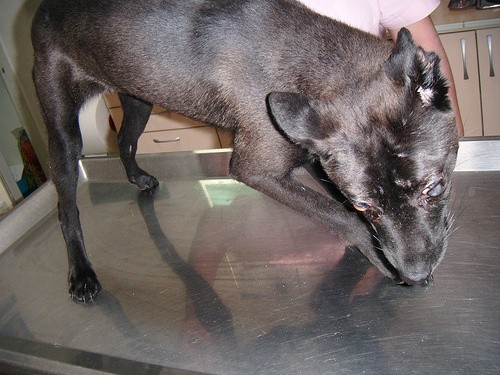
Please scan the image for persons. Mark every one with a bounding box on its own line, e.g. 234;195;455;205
301;0;464;139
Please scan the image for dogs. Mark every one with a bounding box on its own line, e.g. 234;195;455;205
31;0;460;304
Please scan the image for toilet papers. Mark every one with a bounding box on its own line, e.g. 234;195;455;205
78;92;121;155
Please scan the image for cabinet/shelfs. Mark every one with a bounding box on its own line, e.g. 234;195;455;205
102;89;235;153
429;1;500;141
